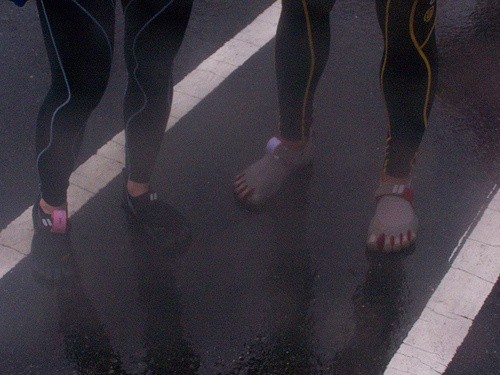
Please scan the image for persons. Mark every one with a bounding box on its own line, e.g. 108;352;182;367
13;0;197;289
232;0;444;260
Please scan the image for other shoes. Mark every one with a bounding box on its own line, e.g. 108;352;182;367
232;135;315;215
366;181;419;252
29;198;82;281
113;181;192;256
